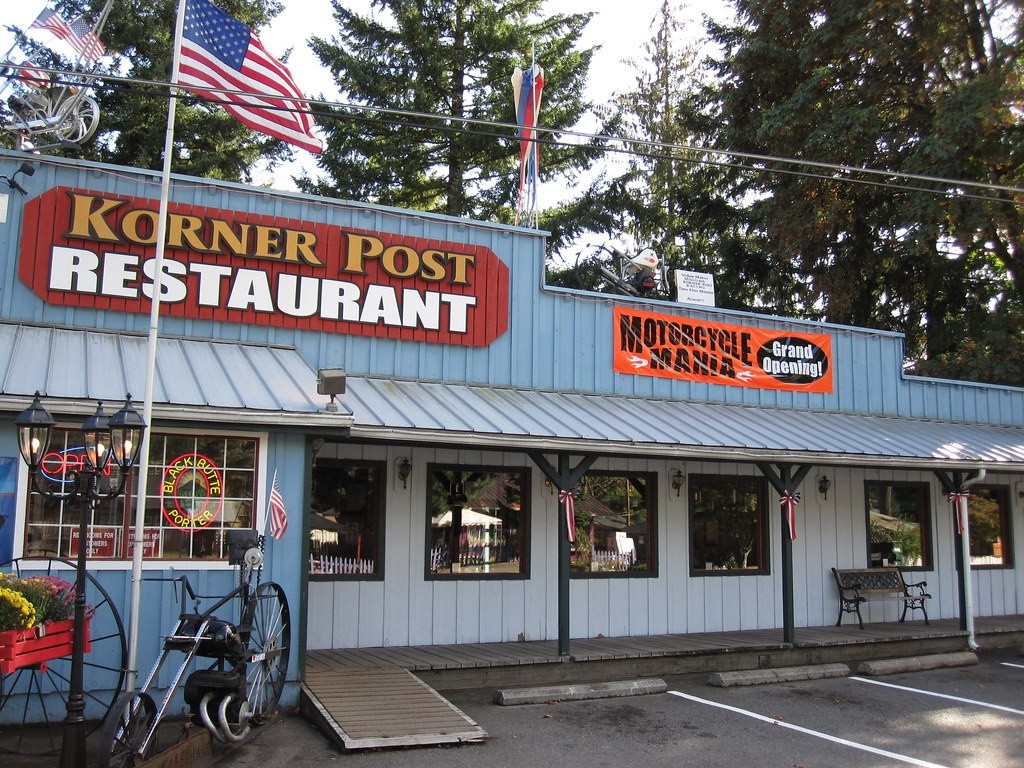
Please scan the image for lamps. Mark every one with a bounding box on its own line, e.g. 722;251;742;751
399;458;412;489
315;366;347;411
226;530;259;566
819;475;832;499
672;468;686;497
312;437;324;466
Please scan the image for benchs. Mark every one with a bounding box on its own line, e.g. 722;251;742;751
832;566;931;629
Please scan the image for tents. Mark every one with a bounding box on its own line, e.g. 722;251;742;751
310;513;338;548
432;508;502;562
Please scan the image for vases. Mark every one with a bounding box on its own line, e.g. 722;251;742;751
0;617;92;674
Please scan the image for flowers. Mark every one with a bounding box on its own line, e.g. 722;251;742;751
0;571;95;630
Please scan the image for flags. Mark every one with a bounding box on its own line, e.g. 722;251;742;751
270;475;288;540
29;6;105;60
170;0;323;155
12;61;52;89
